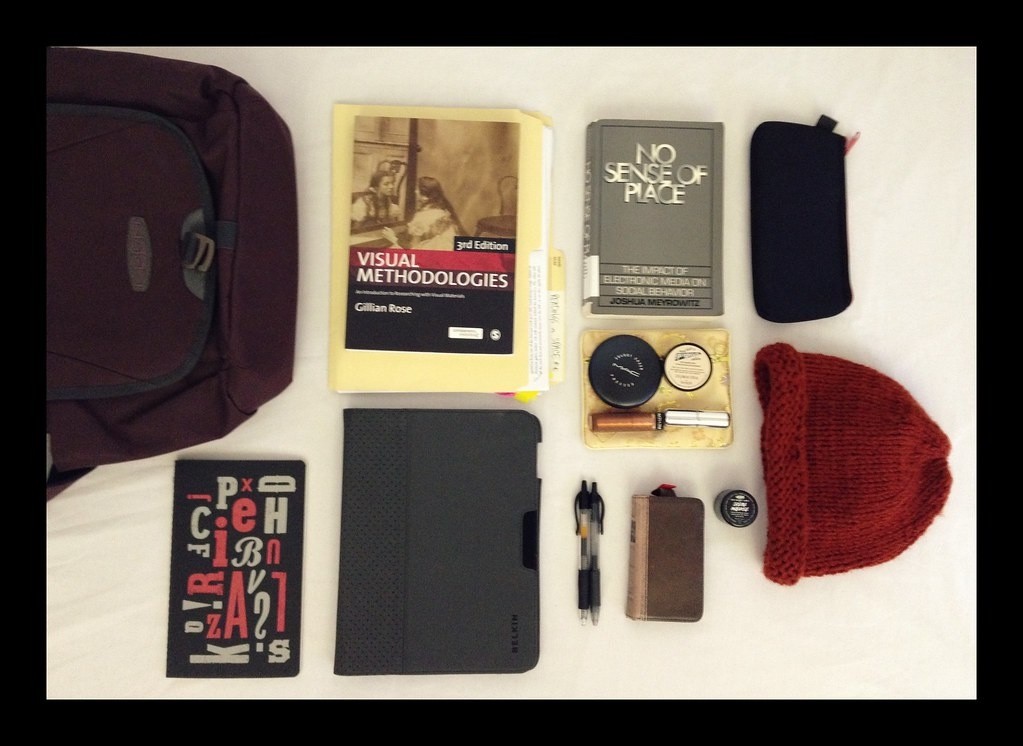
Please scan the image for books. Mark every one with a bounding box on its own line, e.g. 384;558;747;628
331;103;550;395
583;119;725;320
627;486;704;621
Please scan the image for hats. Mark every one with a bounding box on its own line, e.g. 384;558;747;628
753;344;953;584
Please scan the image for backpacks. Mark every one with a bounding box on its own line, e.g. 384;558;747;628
44;46;301;499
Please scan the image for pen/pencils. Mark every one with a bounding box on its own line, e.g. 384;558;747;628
591;482;606;626
574;480;591;627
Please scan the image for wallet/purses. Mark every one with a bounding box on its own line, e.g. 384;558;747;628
749;114;859;324
